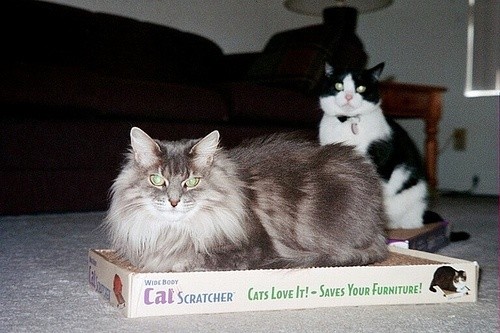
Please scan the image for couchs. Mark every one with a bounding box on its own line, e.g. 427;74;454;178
0;0;368;219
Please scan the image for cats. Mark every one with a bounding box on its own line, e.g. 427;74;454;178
318;60;470;241
88;127;389;270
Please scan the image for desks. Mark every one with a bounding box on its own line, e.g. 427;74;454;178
378;79;447;184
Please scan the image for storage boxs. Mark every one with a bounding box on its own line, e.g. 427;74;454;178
387;221;452;253
88;248;480;319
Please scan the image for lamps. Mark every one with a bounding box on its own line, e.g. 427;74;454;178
283;0;396;28
